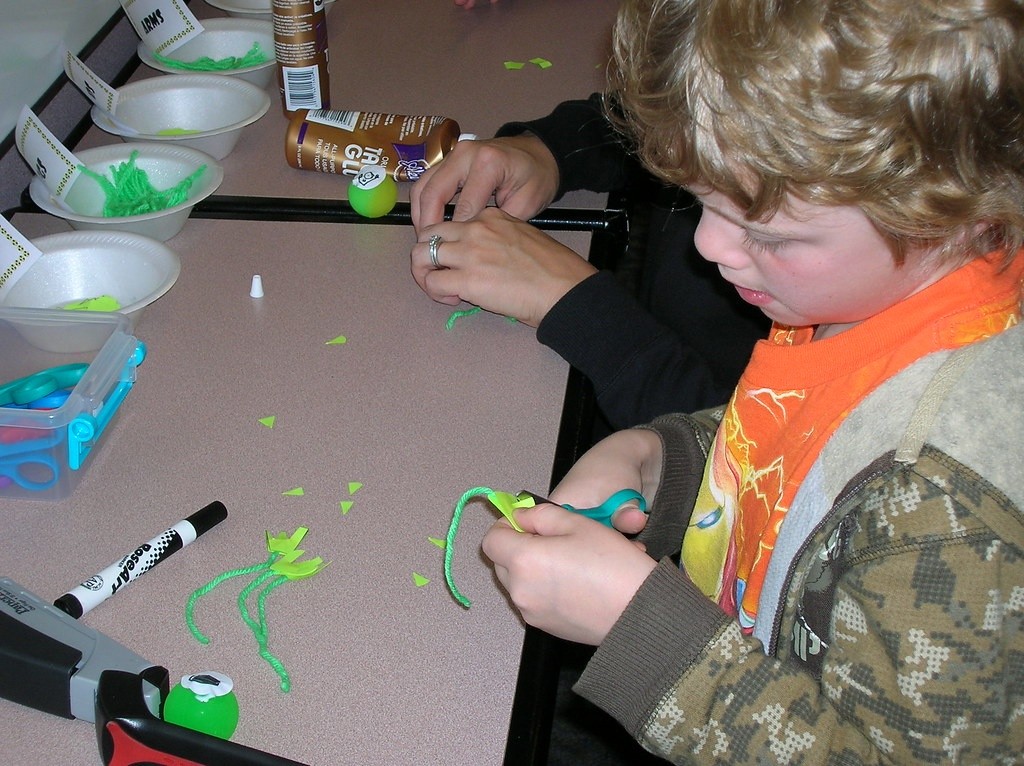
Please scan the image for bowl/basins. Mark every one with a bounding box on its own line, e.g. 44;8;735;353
27;140;224;241
205;0;337;21
0;231;181;353
92;73;271;159
136;16;300;88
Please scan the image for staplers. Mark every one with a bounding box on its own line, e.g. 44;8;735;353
0;577;168;729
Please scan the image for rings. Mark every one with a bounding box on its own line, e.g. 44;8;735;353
429;234;446;270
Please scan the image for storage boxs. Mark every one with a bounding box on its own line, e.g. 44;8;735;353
0;307;148;501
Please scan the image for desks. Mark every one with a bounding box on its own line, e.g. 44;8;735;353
0;0;648;766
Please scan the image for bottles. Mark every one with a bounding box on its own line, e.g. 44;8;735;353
284;107;477;182
266;0;334;113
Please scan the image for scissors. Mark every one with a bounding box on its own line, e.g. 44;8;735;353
0;362;94;492
515;487;648;534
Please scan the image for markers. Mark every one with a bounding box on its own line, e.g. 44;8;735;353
52;500;229;620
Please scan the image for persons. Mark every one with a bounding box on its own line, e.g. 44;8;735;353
411;78;776;429
481;0;1022;763
